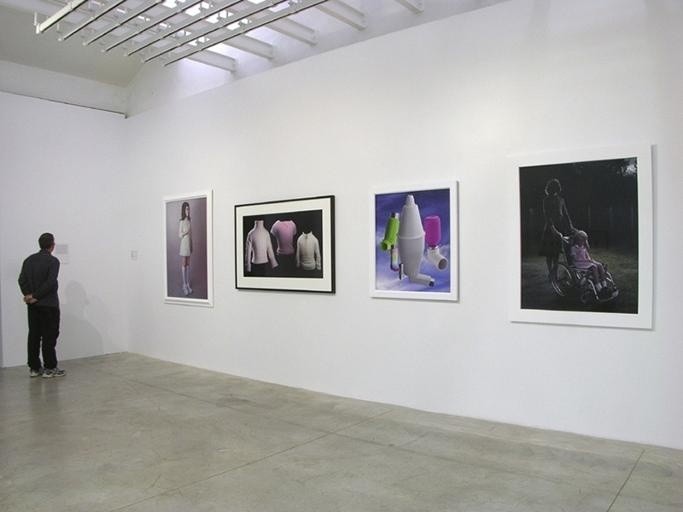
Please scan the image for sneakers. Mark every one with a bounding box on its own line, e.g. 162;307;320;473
182;284;193;296
29;366;67;378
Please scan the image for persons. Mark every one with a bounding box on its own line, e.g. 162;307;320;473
570;231;611;300
18;233;65;378
178;202;193;295
245;220;321;273
538;179;576;282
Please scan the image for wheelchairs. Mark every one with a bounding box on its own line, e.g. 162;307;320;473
548;229;618;306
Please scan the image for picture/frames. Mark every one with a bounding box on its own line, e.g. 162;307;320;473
367;180;461;302
162;191;215;307
234;195;335;293
506;144;655;332
